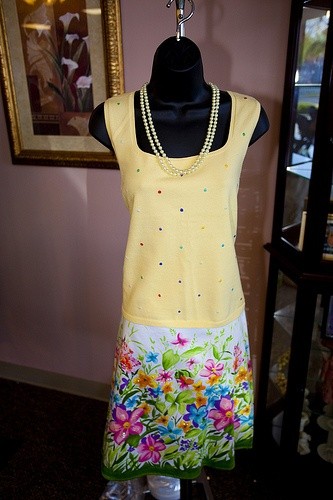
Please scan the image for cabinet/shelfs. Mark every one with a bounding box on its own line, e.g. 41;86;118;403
254;0;333;500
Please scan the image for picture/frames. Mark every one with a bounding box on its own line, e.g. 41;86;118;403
0;0;126;170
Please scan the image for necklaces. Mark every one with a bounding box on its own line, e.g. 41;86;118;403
140;81;220;176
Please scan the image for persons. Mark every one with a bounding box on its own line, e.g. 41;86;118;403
88;36;270;500
